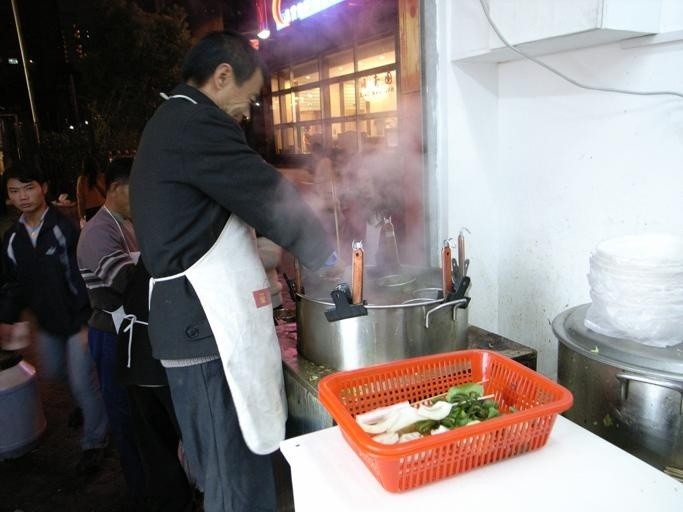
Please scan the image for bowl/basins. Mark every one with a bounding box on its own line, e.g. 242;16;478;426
586;244;683;346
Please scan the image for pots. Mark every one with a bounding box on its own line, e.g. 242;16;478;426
293;261;469;373
549;301;682;483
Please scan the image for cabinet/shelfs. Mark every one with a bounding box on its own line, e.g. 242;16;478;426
450;1;660;63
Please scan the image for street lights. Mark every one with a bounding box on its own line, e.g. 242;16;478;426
8;52;43;146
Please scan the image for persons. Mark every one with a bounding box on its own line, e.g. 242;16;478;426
76;161;164;512
131;30;347;512
3;160;112;473
76;162;107;221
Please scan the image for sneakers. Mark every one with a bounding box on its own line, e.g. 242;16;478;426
67;407;83;427
75;449;98;474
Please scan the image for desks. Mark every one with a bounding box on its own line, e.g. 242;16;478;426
279;391;682;512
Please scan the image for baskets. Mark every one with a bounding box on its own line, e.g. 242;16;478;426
316;349;574;493
